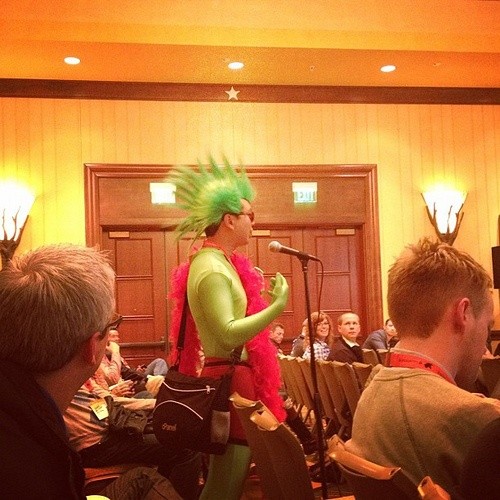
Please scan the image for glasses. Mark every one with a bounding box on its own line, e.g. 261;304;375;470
221;212;254;222
100;312;123;337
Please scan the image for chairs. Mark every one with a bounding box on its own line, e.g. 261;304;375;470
82;348;500;500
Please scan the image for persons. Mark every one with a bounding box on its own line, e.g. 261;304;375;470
362;319;397;350
344;234;500;500
290;318;313;359
268;323;287;356
61;330;169;464
169;195;288;500
0;243;182;500
326;312;363;366
302;311;333;362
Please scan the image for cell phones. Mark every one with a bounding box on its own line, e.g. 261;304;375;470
141;364;148;369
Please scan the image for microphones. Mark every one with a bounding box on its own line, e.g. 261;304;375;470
268;241;321;263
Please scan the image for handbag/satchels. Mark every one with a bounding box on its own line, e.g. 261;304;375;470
104;395;147;435
152;366;232;455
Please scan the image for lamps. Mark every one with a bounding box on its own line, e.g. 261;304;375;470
422;183;468;245
0;179;37;267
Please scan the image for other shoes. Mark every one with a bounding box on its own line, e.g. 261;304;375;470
303;438;328;455
